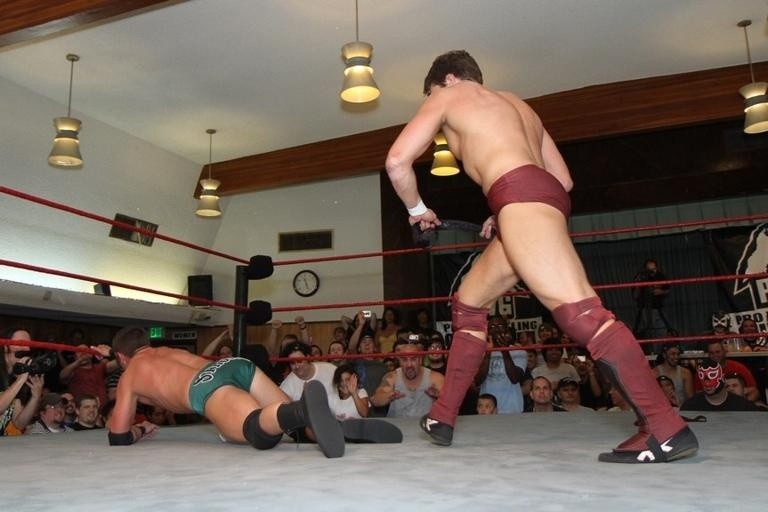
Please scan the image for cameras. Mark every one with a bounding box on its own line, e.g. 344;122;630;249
361;310;372;318
409;334;419;341
13;349;62;376
492;327;517;347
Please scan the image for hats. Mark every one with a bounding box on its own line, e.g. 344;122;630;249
539;324;553;335
39;393;68;409
558;377;578;390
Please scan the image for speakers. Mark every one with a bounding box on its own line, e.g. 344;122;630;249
188;274;213;307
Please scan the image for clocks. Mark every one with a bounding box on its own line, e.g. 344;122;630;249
293;270;319;297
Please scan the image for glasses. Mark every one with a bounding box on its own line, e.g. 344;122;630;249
429;344;443;350
291;343;305;351
68;399;76;403
490;324;504;330
80;405;98;409
46;405;64;411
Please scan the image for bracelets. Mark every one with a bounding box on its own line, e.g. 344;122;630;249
406;201;429;216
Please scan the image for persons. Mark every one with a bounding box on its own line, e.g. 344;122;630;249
385;49;698;465
1;308;768;436
89;325;403;458
631;258;671;328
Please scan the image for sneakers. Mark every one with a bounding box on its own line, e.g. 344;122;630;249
420;413;453;445
599;427;698;464
304;380;345;458
341;417;402;444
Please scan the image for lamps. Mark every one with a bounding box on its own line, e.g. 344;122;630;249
47;53;84;170
430;131;461;179
735;19;768;134
340;0;380;105
194;129;223;218
